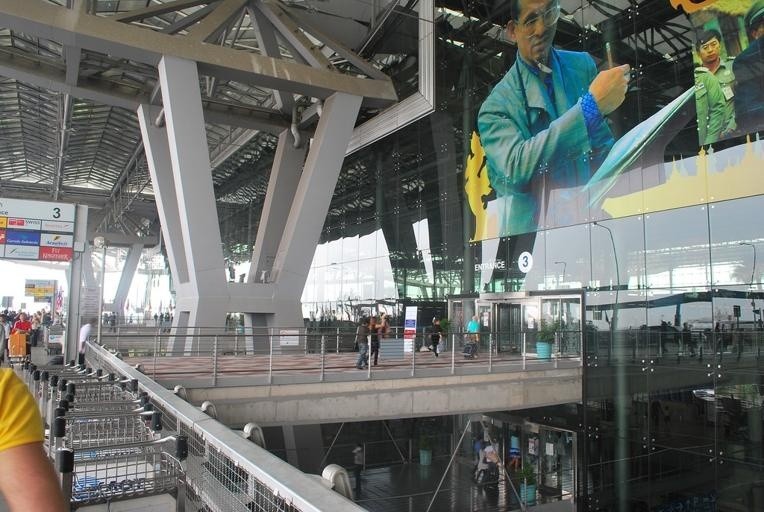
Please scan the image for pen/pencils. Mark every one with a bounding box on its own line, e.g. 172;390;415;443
606;42;613;69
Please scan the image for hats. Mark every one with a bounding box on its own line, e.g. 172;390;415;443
744;1;764;24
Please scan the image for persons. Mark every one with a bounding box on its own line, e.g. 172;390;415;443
462;314;481;360
662;405;673;426
0;307;66;366
353;318;371;370
77;317;98;364
742;0;764;42
101;311;173;334
467;428;521;491
721;411;731;439
369;316;388;366
427;317;442;358
477;1;632;238
650;395;663;426
0;365;69;512
731;34;763;137
693;27;742;143
348;441;364;493
693;65;727;147
655;318;730;358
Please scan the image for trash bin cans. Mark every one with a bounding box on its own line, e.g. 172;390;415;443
744;406;764;445
345;464;362;500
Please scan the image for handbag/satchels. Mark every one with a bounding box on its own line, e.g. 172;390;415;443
437;341;447;354
353;339;359;351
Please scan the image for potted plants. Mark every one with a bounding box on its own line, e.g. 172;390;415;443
517;463;538;506
536;319;565;361
437;318;455;352
419;434;432;466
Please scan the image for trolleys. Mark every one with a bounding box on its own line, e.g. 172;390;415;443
10;329;188;512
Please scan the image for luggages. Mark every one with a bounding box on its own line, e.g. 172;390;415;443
463;340;478;359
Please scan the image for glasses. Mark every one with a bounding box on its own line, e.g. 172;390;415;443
514;0;561;39
